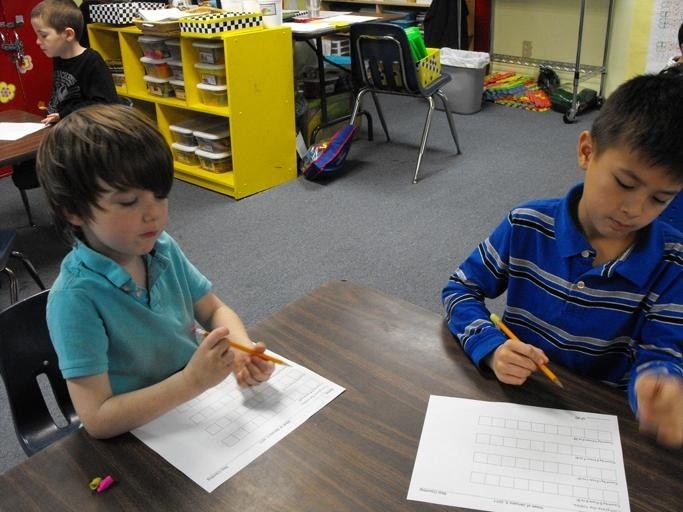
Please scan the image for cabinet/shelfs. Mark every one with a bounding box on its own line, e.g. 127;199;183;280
87;23;297;200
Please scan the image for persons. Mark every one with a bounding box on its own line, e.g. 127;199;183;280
34;101;276;441
662;22;683;76
439;73;683;452
30;1;120;128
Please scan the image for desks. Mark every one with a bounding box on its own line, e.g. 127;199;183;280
0;276;682;510
0;109;58;167
281;9;406;146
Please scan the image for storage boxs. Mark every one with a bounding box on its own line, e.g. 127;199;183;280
137;35;232;174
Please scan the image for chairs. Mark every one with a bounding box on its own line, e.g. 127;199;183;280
0;225;46;305
0;288;82;458
349;22;461;183
11;94;134;226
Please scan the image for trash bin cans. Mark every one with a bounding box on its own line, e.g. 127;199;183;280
432;47;490;114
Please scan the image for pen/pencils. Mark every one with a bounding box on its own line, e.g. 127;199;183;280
490;314;564;390
190;328;293;367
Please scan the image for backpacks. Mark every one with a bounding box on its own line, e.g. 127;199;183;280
300;123;358;185
